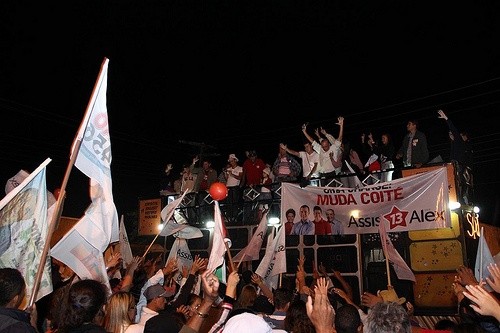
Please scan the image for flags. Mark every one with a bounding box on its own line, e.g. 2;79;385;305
0;57;497;309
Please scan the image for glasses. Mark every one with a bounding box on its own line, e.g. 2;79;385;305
130;304;137;308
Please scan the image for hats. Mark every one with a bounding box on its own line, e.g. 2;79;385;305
228;153;238;162
143;283;175;299
263;168;270;175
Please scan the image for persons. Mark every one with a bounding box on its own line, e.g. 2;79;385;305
0;111;500;333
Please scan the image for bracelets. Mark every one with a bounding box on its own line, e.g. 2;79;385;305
195;308;208;318
105;265;109;271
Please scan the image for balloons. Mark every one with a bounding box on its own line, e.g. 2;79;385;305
54;189;61;201
210;182;228;200
